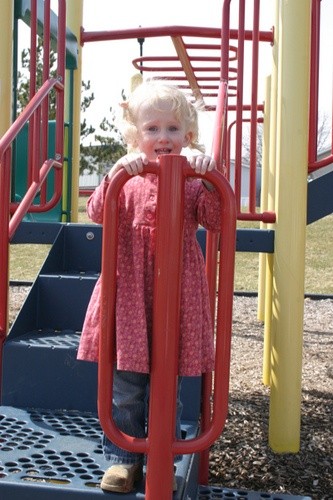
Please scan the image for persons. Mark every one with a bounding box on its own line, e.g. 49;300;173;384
76;80;224;493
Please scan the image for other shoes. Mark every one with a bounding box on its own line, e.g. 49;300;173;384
173;477;177;491
101;464;134;493
